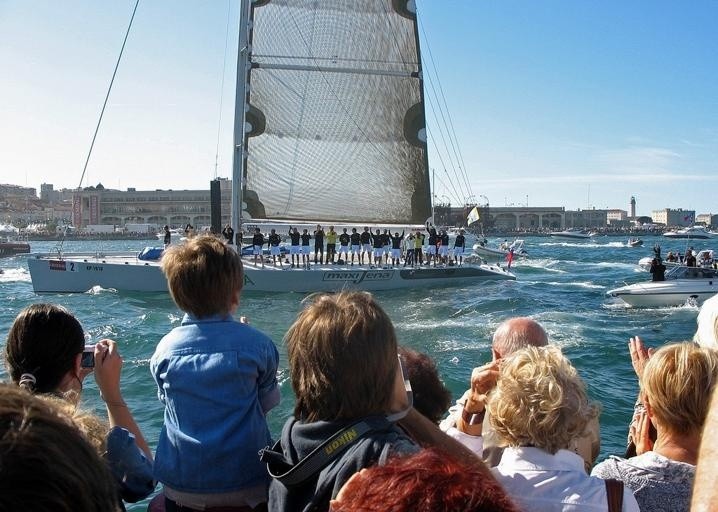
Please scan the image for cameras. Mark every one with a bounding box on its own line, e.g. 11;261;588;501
80;345;102;368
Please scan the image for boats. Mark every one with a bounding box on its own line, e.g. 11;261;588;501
629;239;643;247
548;228;592;242
607;265;718;309
664;227;715;239
473;238;526;258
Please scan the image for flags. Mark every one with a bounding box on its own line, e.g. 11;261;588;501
466;208;479;226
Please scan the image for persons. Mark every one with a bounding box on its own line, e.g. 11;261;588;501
258;291;496;512
650;246;696;281
288;224;465;269
438;317;548;433
185;224;194;233
0;385;126;512
444;346;640;512
5;302;158;512
150;233;280;512
163;225;171;250
252;228;282;268
590;294;718;512
328;448;518;512
223;224;234;248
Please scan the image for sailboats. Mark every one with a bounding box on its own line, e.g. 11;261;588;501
25;0;516;296
